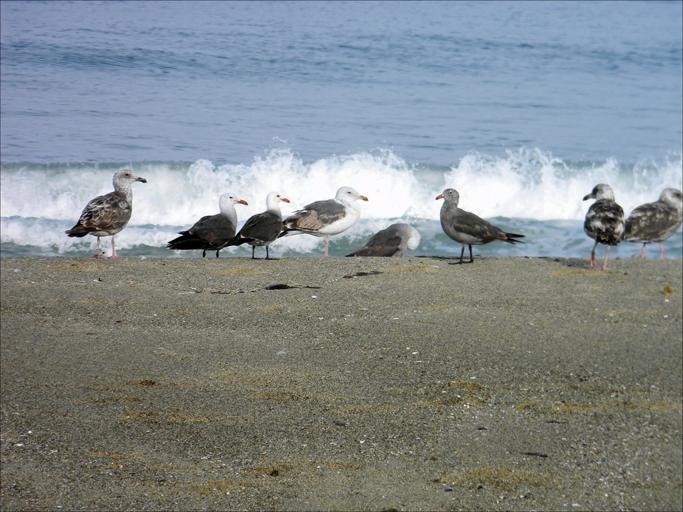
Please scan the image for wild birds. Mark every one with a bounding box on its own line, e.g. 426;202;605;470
582;182;625;273
345;223;422;256
278;186;369;257
225;191;289;260
65;168;147;261
624;188;682;261
164;192;248;258
435;187;526;265
